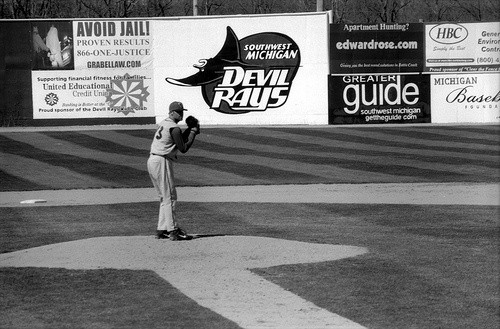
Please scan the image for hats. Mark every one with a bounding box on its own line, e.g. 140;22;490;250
168;101;188;112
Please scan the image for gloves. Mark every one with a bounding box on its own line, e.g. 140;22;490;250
185;119;200;134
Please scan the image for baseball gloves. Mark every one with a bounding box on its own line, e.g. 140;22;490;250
186;116;201;135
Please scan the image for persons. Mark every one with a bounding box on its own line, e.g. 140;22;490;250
146;101;200;241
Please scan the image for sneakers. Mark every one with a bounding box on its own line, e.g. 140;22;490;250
169;227;193;241
155;230;171;239
